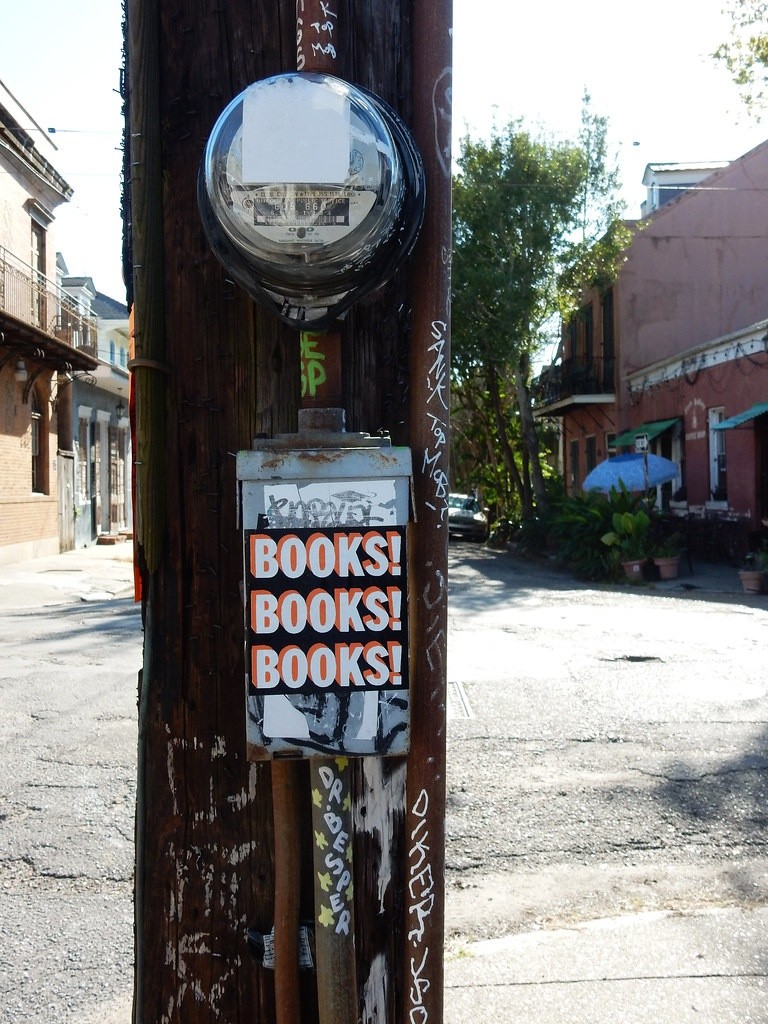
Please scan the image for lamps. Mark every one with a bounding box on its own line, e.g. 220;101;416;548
13;358;27;381
116;388;126;419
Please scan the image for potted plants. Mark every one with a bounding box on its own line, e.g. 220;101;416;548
601;510;656;584
654;532;681;579
737;551;768;595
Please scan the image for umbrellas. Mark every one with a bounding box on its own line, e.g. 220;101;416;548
582;453;678;494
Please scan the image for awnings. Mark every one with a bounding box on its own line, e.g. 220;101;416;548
610;419;678;446
710;402;768;431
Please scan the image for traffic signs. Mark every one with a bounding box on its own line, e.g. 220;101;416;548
635;433;649;454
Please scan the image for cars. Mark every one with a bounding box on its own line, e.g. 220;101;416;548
449;493;491;543
